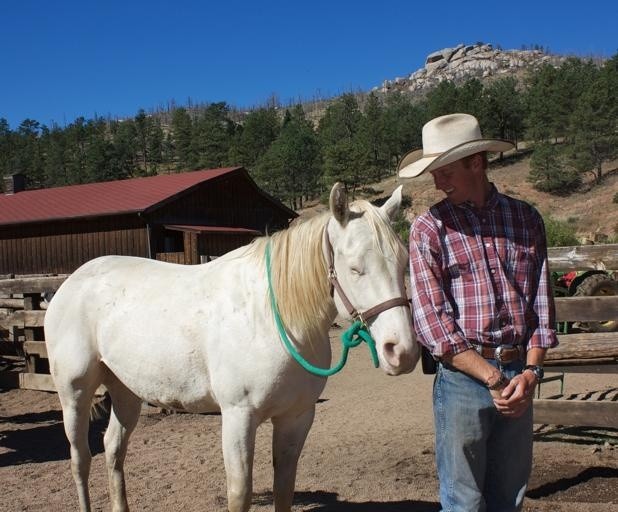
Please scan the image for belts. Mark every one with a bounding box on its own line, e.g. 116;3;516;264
477;343;528;361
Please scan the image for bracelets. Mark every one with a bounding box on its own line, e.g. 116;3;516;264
487;372;505;390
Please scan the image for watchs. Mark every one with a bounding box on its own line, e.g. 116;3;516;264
521;365;544;382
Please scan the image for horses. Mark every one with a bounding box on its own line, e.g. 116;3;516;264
42;182;421;512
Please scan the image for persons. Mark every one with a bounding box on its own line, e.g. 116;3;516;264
398;114;558;512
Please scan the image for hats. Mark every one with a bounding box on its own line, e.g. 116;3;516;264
396;112;516;181
